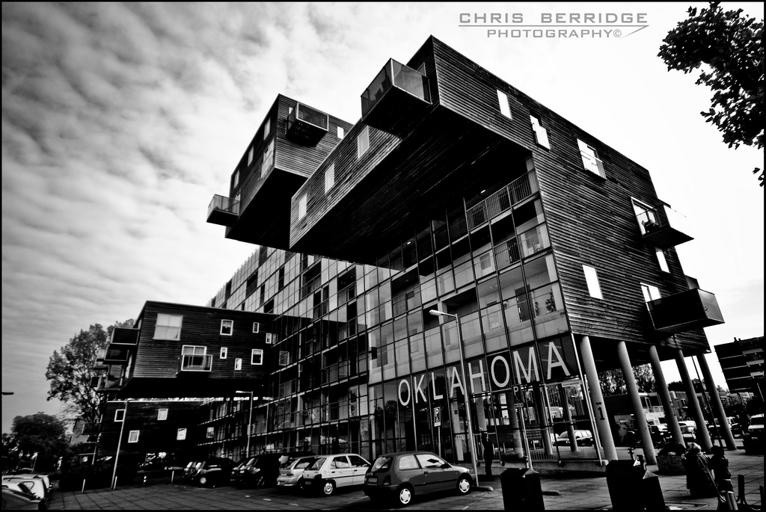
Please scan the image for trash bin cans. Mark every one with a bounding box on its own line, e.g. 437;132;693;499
500;467;545;512
605;460;646;512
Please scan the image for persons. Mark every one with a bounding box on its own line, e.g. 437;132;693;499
480;433;496;476
260;449;289;489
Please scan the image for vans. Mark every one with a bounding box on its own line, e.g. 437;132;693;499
554;429;594;447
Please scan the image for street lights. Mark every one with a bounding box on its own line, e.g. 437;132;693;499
236;390;253;458
429;309;494;492
110;397;135;490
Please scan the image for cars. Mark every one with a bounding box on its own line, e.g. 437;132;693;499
1;473;54;510
708;416;740;433
363;450;474;506
182;452;371;497
747;412;765;437
